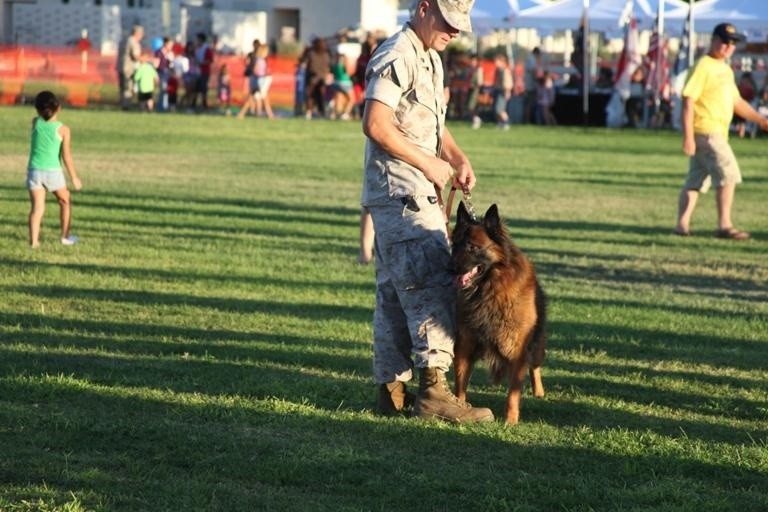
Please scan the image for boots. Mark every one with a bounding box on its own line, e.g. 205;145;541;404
413;368;495;425
377;382;417;418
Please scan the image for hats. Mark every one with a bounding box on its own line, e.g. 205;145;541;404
714;23;746;42
436;0;476;34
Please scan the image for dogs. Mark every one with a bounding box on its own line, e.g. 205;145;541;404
448;199;549;429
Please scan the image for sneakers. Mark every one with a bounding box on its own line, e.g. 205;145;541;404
60;235;80;246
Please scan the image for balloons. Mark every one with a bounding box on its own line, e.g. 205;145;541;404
151;37;163;51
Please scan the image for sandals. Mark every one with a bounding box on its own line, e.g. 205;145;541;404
718;228;751;241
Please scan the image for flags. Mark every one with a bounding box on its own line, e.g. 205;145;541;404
571;8;585;73
617;12;637;85
645;13;670;93
670;16;689;101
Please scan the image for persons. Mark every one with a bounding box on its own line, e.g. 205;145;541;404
135;54;159;111
118;25;144;110
329;54;356;121
362;0;495;424
734;72;755;138
218;64;230;104
522;46;540;123
245;40;263;117
535;71;557;126
626;66;646;128
448;49;481;132
366;30;378;54
190;33;213;107
78;28;90;73
168;44;189;107
154;38;174;112
182;42;195;106
675;24;768;241
490;54;513;130
296;38;330;120
295;58;308;117
27;91;82;249
237;44;275;120
758;84;768;118
